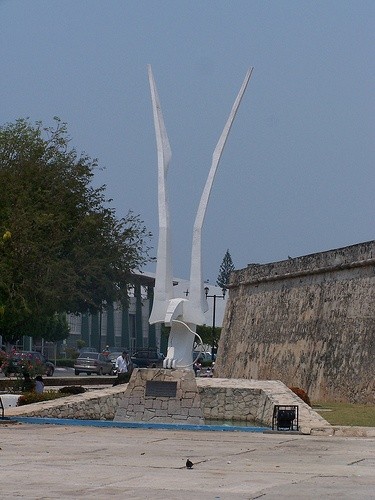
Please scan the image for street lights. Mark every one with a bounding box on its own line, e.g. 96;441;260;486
204;286;227;378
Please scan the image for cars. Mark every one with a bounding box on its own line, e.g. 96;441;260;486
193;351;214;370
72;351;114;376
130;351;165;368
3;350;55;376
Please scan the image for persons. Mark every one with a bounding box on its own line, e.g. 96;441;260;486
102;345;112;354
32;375;45;393
115;352;129;387
193;361;202;375
